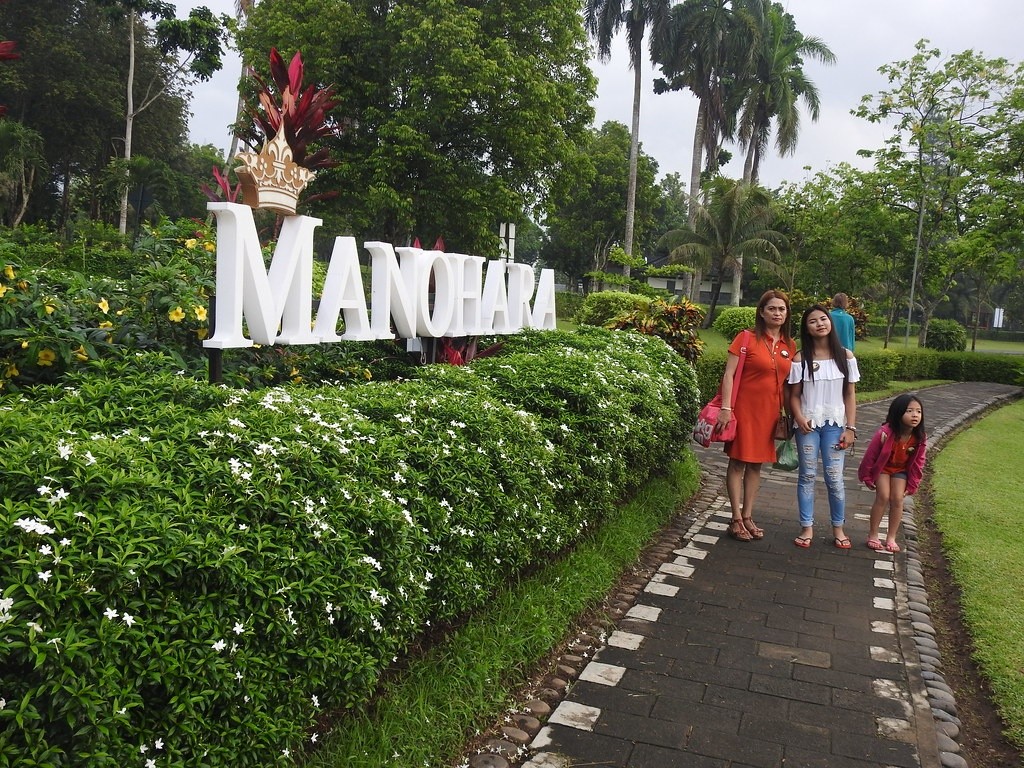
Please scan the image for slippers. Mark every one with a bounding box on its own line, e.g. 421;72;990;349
834;537;852;549
795;536;811;548
886;540;900;552
867;535;883;550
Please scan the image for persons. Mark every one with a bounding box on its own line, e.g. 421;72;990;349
858;394;927;552
787;303;860;549
713;289;796;541
830;292;855;354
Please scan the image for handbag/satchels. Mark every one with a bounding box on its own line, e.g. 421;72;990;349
773;415;795;441
772;439;799;471
694;393;736;448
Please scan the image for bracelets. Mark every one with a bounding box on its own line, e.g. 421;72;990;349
846;426;858;440
721;407;731;411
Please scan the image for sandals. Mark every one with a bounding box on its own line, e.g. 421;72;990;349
743;517;765;539
727;518;753;542
